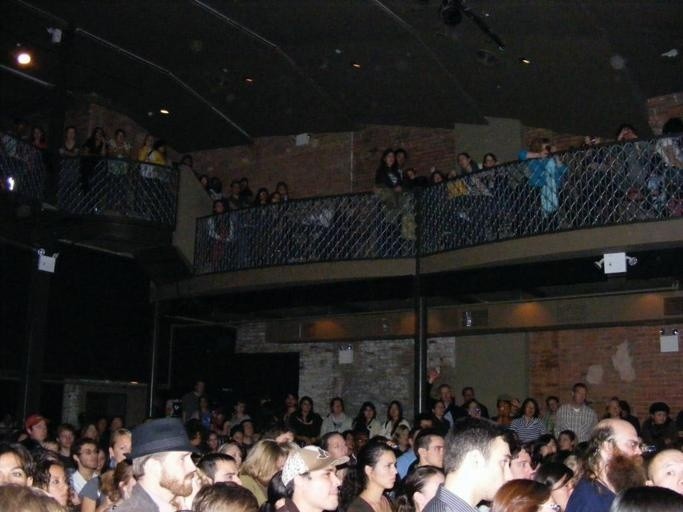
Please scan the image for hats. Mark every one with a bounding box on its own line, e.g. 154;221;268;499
122;417;200;460
281;445;351;486
26;413;51;428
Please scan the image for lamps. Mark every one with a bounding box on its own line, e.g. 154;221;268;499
592;252;638;275
658;327;681;352
338;343;354;364
36;248;60;274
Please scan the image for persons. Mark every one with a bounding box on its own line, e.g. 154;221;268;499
5;116;681;272
0;367;683;512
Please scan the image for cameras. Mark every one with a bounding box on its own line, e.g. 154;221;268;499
473;407;481;415
545;146;551;151
641;445;657;453
